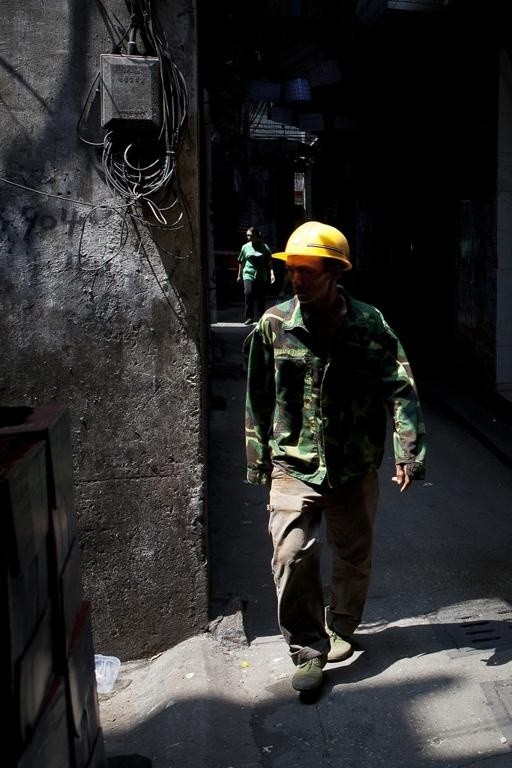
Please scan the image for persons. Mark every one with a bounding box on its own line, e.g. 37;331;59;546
243;221;428;691
237;225;275;325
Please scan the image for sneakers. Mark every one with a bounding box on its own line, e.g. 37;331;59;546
292;630;354;691
245;319;253;325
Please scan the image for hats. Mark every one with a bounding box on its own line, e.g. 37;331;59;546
270;221;352;271
